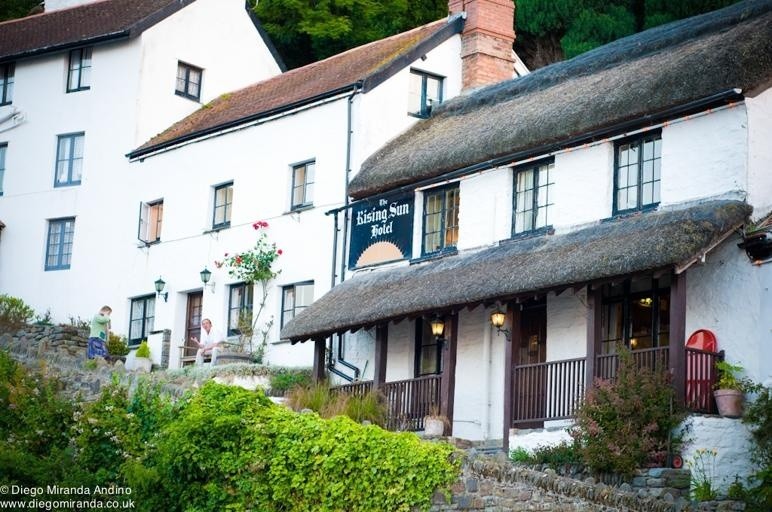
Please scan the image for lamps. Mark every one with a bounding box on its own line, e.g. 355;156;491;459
200;264;216;294
154;274;168;303
431;313;449;348
490;306;511;342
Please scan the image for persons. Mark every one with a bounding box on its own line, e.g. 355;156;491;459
87;305;112;362
190;318;225;368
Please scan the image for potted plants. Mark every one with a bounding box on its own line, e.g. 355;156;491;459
127;338;153;373
711;358;752;418
